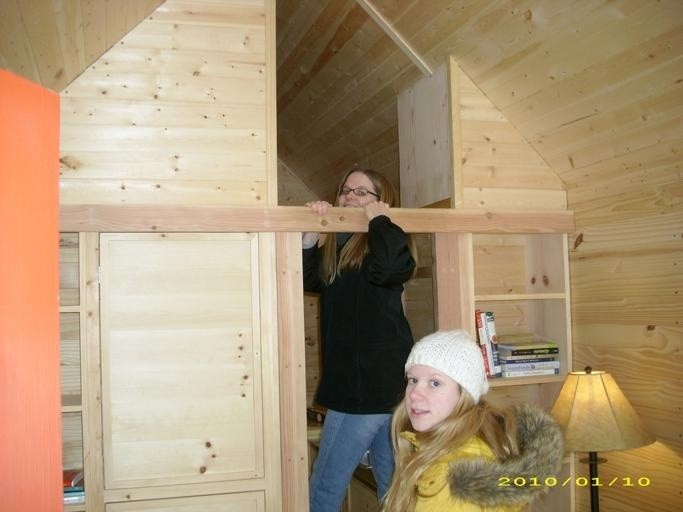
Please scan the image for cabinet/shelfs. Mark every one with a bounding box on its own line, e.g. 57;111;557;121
457;231;590;512
56;230;288;511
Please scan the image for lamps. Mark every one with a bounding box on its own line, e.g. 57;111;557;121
550;366;656;512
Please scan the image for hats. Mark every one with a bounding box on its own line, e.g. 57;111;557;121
403;327;490;406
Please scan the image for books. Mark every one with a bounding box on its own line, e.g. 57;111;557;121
475;310;561;378
62;471;84;503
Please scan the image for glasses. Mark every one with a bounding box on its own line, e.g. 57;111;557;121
340;186;380;198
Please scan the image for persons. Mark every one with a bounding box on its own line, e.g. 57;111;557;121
301;166;413;512
382;330;563;512
